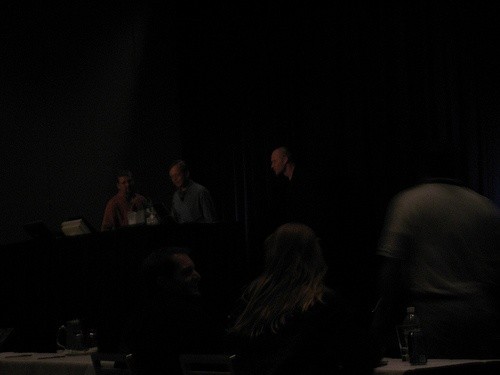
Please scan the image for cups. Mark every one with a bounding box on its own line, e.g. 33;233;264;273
87;328;97;347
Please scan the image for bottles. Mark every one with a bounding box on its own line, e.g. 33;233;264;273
402;307;422;361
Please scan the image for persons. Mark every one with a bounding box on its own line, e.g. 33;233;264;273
102;171;157;232
376;135;500;358
257;148;313;205
114;246;231;375
224;223;382;375
169;161;218;224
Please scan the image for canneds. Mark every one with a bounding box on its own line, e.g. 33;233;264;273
407;328;427;365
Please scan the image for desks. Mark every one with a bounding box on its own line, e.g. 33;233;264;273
0;347;116;375
373;355;500;375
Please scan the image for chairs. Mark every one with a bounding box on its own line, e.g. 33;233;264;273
178;352;247;375
91;352;139;375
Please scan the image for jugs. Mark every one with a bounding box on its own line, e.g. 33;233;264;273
57;320;83;351
